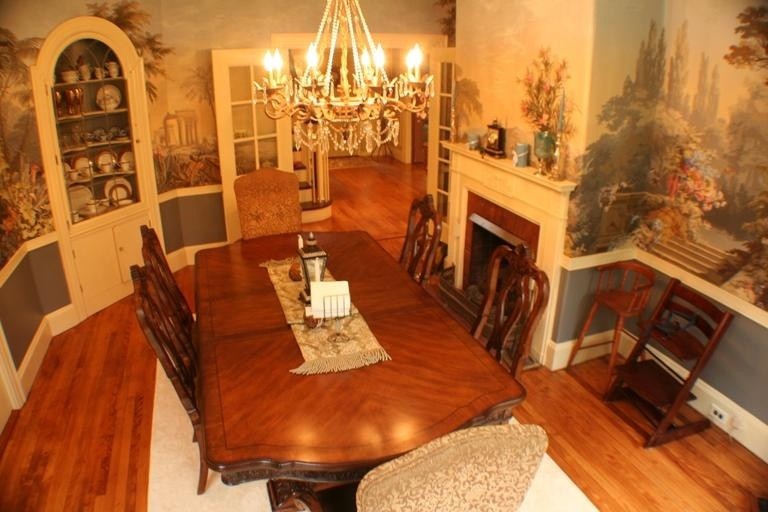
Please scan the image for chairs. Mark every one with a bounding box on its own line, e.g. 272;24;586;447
567;263;654;394
605;278;736;446
472;243;550;383
131;266;208;495
398;194;441;290
308;424;548;512
140;225;197;353
235;168;303;241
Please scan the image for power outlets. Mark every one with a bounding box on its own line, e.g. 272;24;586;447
708;404;728;422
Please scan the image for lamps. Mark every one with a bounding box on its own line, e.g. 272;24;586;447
255;0;435;155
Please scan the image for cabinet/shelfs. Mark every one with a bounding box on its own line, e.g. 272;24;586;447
30;13;156;225
70;211;171;317
